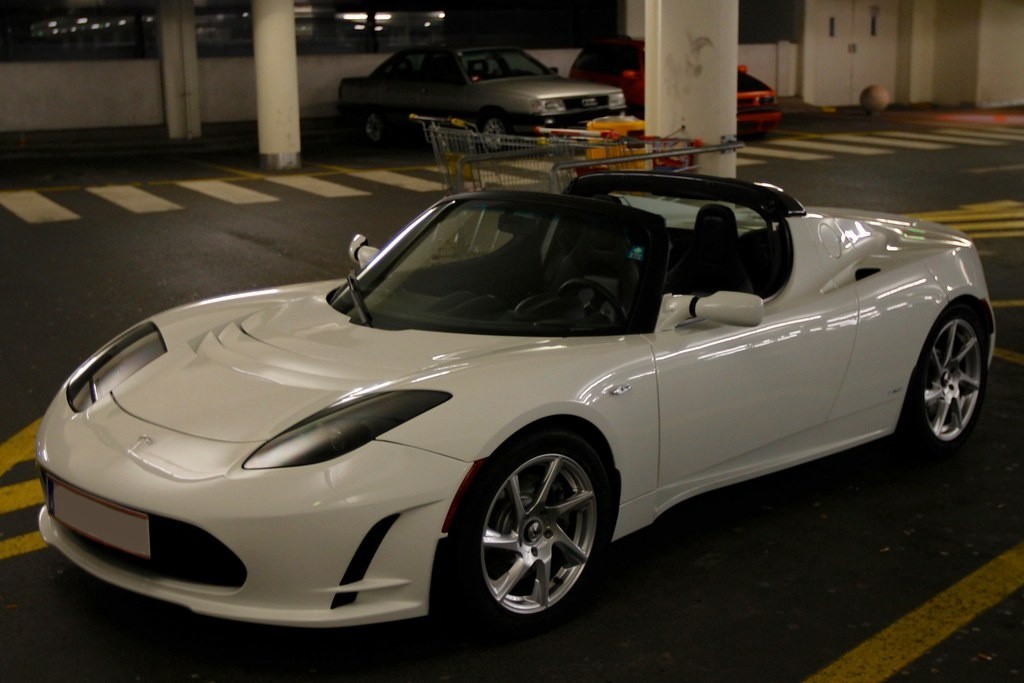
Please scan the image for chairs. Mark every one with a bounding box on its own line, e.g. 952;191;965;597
541;192;640;323
661;202;756;297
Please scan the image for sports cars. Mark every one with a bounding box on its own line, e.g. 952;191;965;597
36;172;997;646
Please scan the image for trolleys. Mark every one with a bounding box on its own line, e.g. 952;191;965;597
409;113;744;194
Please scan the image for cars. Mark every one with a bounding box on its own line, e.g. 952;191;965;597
570;36;782;140
338;44;626;153
